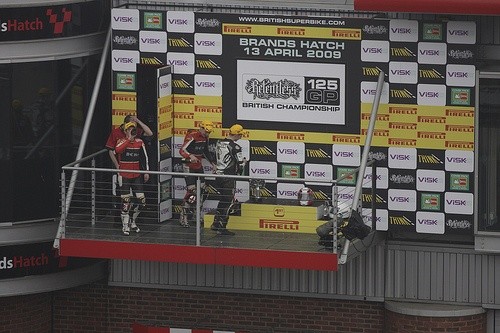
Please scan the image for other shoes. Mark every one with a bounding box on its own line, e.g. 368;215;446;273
192;216;203;224
211;224;219;230
179;218;190;228
131;222;140;232
216;229;235;236
122;226;130;235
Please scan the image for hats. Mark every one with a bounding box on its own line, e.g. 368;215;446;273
229;124;245;135
199;120;214;133
124;122;136;131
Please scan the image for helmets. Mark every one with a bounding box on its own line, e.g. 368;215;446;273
298;187;313;206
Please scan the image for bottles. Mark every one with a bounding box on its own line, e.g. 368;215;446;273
180;159;202;166
131;129;137;136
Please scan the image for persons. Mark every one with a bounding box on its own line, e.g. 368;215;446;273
179;119;215;230
115;121;150;237
9;86;59;146
105;115;153;228
210;123;248;236
